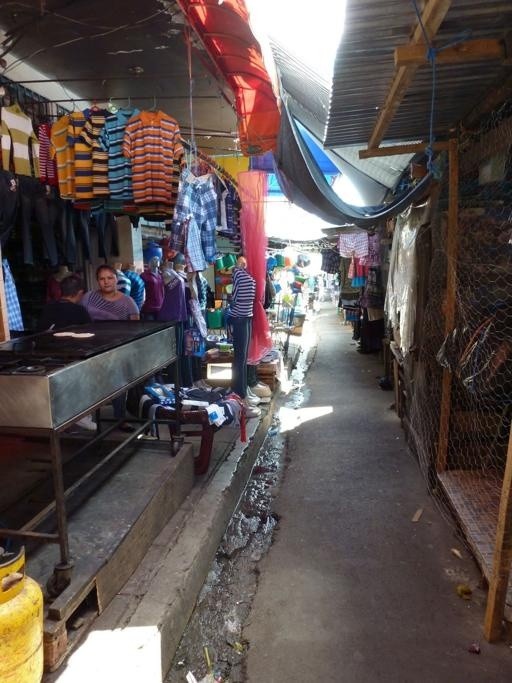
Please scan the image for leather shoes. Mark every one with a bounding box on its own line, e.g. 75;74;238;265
118;421;137;433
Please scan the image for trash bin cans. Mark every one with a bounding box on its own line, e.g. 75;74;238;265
288;312;305;336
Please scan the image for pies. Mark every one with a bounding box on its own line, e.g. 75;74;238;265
73;332;95;338
53;331;75;336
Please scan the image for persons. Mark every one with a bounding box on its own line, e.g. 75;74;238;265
82;262;141;433
126;262;146;310
163;260;187;391
139;253;166;386
35;272;99;432
111;261;132;296
229;255;256;399
196;269;208;319
175;263;195;387
44;263;84;302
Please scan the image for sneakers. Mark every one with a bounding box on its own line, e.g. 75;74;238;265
74;414;98;431
